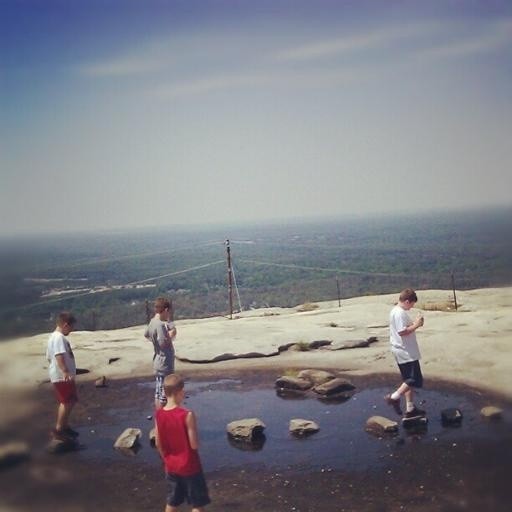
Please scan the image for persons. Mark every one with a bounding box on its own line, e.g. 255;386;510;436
154;373;210;512
144;298;177;409
46;311;79;437
384;288;426;416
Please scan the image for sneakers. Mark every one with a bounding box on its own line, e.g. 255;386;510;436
50;429;75;442
405;407;426;418
64;427;79;438
384;393;403;416
